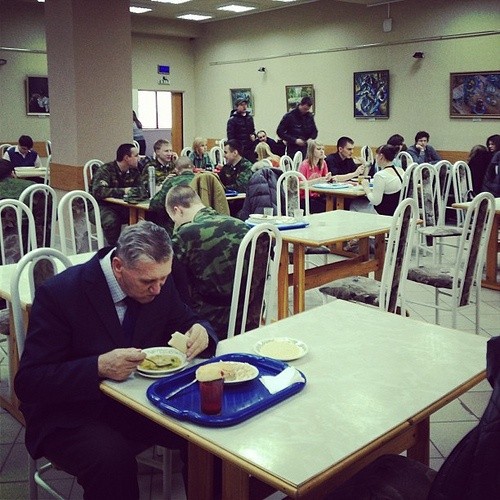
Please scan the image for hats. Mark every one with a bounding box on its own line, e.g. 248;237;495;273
234;98;248;107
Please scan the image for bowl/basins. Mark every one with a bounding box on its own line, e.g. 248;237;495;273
14;166;34;172
249;214;276;224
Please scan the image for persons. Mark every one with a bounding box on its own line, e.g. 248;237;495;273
13;221;222;500
133;111;146;156
1;134;49;186
187;137;214;172
165;186;263;340
84;144;150;247
226;97;255;157
297;130;500;254
328;336;500;500
277;97;318;161
247;130;277;155
0;159;53;266
137;139;175;177
251;141;280;168
144;156;195;227
211;138;253;195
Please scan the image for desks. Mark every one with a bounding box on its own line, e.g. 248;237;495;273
452;198;500;290
262;209;423;322
301;181;368;259
104;191;250;228
16;169;50;178
0;252;101;427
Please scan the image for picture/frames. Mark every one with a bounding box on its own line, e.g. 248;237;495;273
230;88;255;116
285;85;315;116
26;75;50;116
353;70;389;119
449;71;500;119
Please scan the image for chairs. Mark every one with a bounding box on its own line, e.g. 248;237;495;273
0;135;494;500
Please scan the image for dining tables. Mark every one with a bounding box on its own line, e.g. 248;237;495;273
102;297;489;500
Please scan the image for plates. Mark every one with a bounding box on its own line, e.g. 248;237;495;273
135;347;187;378
254;337;308;362
197;361;259;386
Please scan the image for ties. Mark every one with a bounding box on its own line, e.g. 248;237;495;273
120;295;142;343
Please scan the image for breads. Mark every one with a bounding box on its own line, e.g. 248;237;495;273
168;331;189;355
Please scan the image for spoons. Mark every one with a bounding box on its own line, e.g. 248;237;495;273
144;357;171;367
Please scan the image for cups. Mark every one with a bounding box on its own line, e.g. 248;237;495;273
200;379;224;417
294;209;304;221
264;207;273;218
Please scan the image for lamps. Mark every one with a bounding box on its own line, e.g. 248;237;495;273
259;68;266;72
413;52;424;58
0;58;7;65
